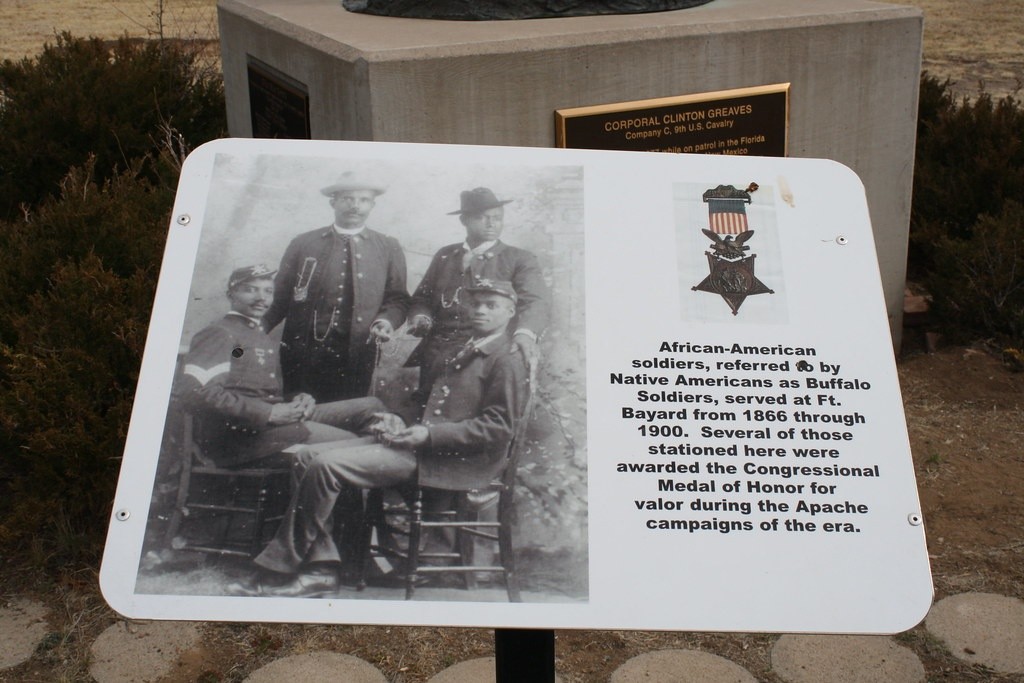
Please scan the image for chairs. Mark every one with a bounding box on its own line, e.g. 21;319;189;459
166;347;308;590
353;329;538;601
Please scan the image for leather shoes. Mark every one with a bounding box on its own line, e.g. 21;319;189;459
223;572;276;596
386;571;430;588
272;573;340;598
437;490;500;526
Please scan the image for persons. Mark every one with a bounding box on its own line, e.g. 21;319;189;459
179;166;548;595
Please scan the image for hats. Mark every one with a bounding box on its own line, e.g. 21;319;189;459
466;280;518;306
320;170;388;198
228;265;278;289
445;187;516;217
